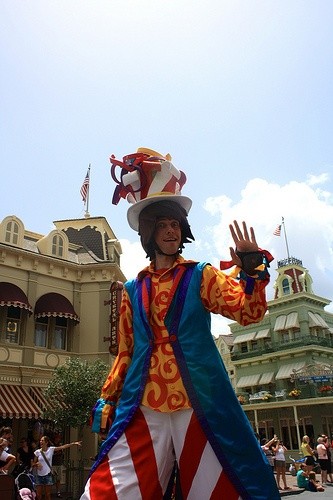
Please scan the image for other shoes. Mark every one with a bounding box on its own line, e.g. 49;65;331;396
278;487;283;490
284;486;289;490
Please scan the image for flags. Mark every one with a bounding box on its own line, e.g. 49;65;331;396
80;166;91;205
272;224;281;236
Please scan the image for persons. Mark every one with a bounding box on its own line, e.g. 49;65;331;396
0;426;82;500
78;200;281;500
272;439;290;490
261;433;280;473
289;435;333;492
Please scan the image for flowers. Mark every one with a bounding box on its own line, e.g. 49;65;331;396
238;396;246;402
321;386;333;392
262;394;272;399
289;390;301;396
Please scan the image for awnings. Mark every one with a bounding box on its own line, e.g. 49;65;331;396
30;386;95;421
35;293;80;326
0;384;45;422
259;372;275;386
236;374;261;388
313;361;333;371
233;332;256;343
285;313;300;329
325;328;333;335
255;329;271;340
307;311;322;328
276;361;307;379
274;315;287;333
0;282;34;318
314;314;329;329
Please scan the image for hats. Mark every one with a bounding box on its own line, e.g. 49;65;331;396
108;147;192;232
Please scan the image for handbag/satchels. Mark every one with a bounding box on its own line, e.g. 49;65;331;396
51;468;61;485
